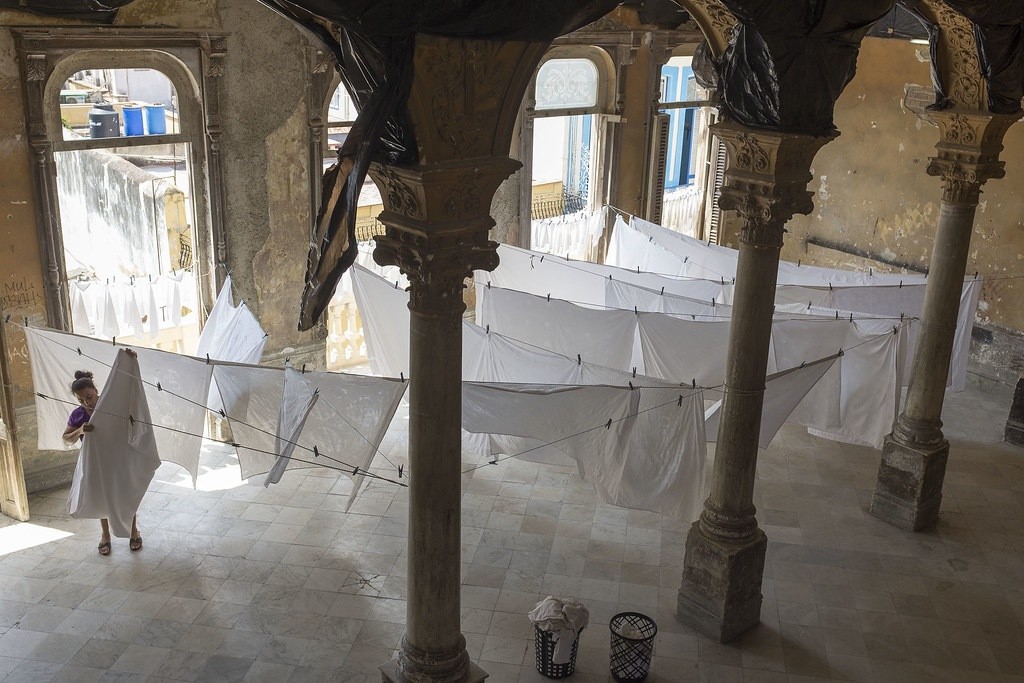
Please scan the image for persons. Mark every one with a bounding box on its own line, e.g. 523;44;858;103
62;348;142;555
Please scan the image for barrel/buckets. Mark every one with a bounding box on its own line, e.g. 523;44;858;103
124;108;144;136
89;105;120;138
145;105;167;135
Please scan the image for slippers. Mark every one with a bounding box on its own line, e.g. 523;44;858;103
99;539;111;554
130;531;142;550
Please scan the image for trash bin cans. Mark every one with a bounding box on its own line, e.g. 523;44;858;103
145;104;166;134
123;106;144;136
